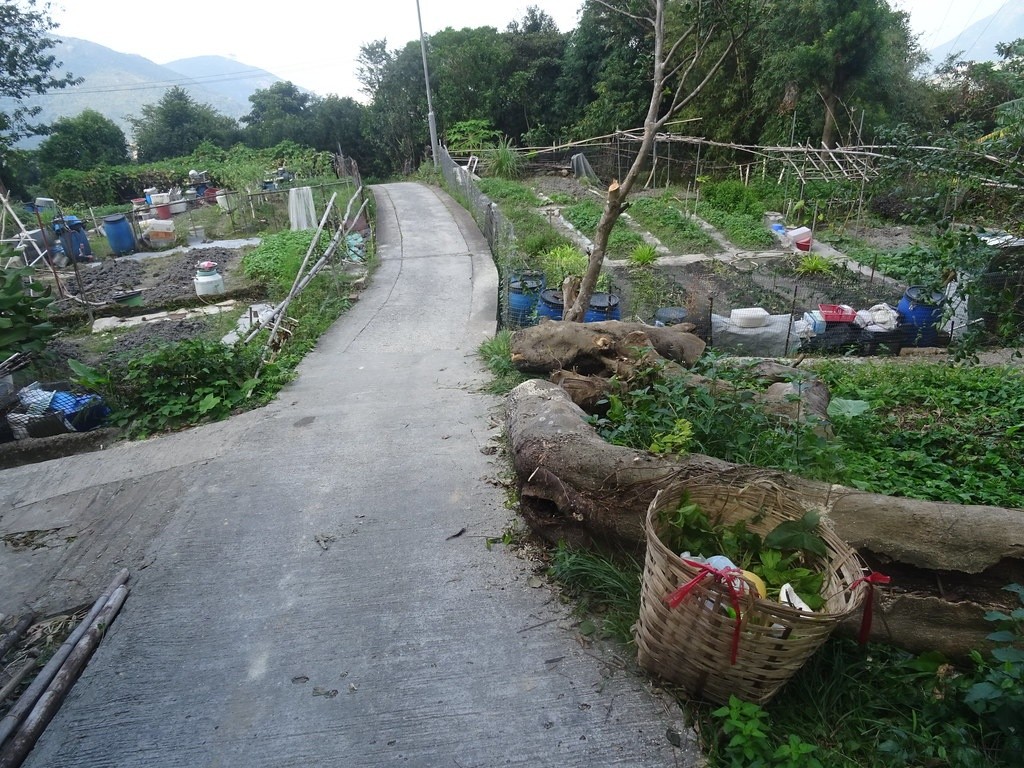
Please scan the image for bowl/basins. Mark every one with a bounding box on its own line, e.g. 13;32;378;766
195;262;218;273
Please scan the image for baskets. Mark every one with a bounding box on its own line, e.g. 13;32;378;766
629;468;882;710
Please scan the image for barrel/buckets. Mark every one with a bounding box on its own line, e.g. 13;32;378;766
22;181;241;265
897;286;945;345
507;267;621;327
193;270;225;297
